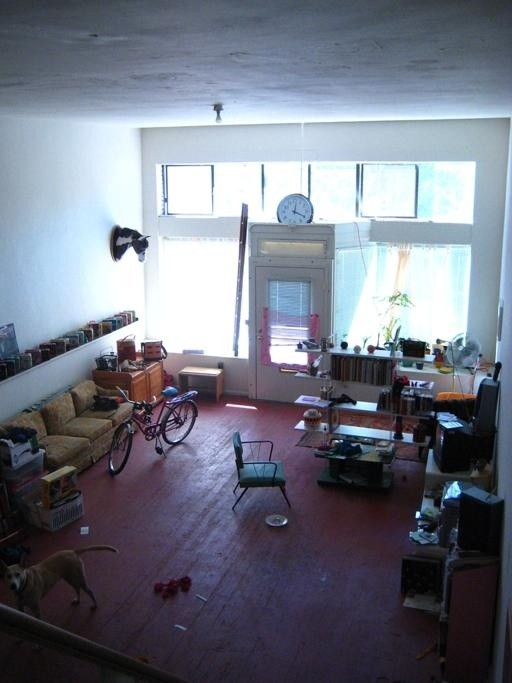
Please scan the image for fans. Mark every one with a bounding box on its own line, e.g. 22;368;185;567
446;332;480;392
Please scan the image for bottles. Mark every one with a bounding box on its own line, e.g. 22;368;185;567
434;352;443;369
321;337;327;352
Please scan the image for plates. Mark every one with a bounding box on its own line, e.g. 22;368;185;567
264;514;289;528
439;366;454;373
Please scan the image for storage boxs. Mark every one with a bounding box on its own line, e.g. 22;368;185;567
0;448;46;493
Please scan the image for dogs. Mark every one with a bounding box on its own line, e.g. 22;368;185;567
0;544;119;621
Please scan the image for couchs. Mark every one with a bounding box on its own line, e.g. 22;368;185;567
0;379;139;484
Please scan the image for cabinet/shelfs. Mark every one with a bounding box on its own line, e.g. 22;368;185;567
92;356;164;417
291;342;486;456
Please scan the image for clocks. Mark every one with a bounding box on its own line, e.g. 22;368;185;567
276;194;313;225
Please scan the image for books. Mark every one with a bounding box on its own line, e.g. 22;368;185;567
374;387;434;418
399;553;442;601
329;354;399;387
1;308;137;380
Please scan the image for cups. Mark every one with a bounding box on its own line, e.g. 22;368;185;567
415;362;424;369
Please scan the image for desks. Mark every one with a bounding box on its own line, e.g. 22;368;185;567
315;444;395;492
178;366;224;402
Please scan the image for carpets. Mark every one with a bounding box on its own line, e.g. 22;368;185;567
295;411;429;463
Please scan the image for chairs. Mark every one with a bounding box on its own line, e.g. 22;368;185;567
232;431;292;509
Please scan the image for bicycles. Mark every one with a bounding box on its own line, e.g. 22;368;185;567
108;382;199;476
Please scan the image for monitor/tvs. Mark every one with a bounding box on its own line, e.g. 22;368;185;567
433;418;482;473
